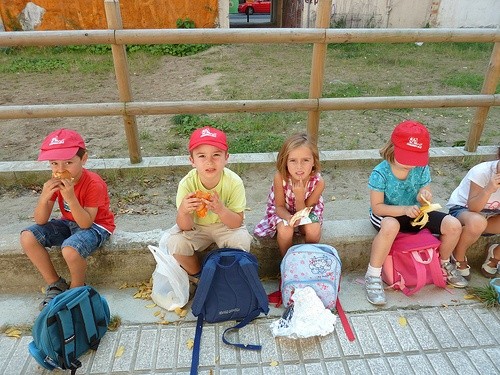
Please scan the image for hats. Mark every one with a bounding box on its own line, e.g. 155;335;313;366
390;120;430;166
38;128;86;161
188;126;227;152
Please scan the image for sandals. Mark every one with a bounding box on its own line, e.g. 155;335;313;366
39;276;70;312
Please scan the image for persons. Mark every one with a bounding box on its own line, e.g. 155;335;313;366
20;128;116;311
166;126;253;310
364;121;469;306
254;134;324;303
446;146;500;280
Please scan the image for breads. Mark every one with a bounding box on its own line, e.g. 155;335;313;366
193;190;211;217
51;170;71;184
290;207;314;227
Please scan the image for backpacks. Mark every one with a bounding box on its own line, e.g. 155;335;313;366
28;282;110;375
381;228;447;296
190;248;270;375
271;284;336;339
278;243;342;313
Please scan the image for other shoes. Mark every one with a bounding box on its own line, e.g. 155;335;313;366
480;242;499;278
450;252;471;281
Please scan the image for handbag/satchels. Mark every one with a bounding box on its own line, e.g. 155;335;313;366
146;229;189;311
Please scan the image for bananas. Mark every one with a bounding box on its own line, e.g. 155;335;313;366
411;195;441;229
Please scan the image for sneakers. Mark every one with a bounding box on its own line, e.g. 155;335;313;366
440;261;468;288
363;274;388;304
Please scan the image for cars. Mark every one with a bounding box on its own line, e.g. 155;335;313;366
237;0;271;15
